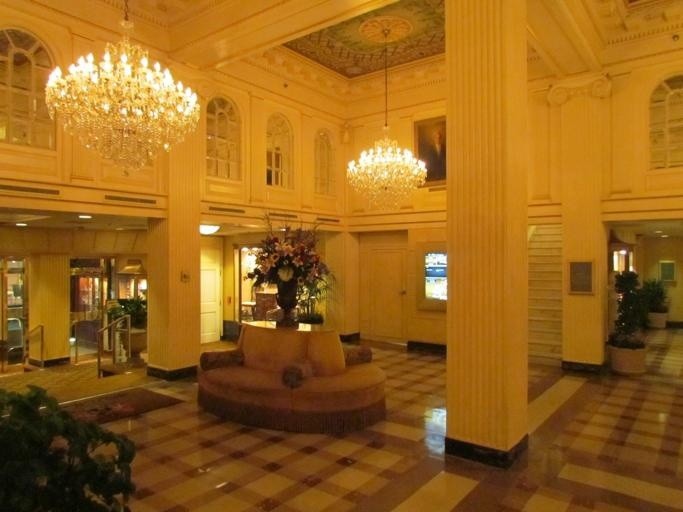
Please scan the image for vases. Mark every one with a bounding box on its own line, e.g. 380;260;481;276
274;278;299;327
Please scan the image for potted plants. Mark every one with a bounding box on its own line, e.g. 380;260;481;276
605;271;670;376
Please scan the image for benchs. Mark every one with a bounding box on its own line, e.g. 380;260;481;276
196;320;388;434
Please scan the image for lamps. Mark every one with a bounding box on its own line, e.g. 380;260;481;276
43;1;202;179
199;224;220;235
346;30;428;214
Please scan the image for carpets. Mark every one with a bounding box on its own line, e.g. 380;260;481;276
39;387;187;426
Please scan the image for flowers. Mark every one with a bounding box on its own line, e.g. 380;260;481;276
243;208;337;305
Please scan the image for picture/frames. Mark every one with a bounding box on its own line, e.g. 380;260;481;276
413;114;447;188
